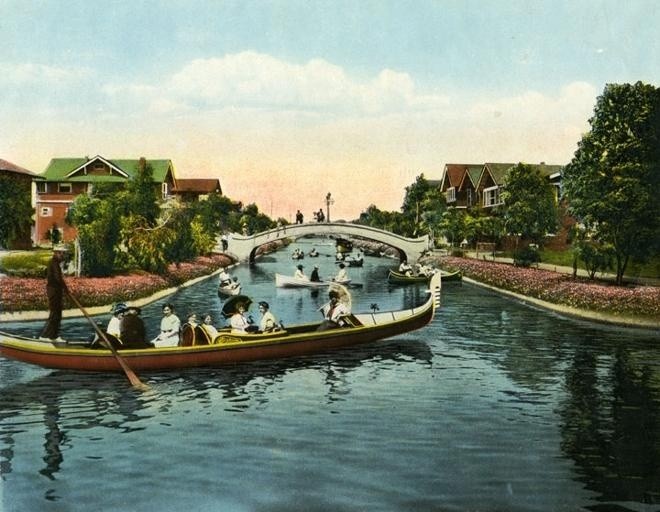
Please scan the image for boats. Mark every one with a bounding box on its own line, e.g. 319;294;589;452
214;280;243;295
274;273;363;290
0;272;441;373
292;237;384;266
386;268;463;284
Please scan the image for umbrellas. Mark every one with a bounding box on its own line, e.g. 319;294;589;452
220;295;253;319
316;281;353;313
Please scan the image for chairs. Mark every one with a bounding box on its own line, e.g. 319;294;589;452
106;333;123;349
195;324;212;345
181;323;194;346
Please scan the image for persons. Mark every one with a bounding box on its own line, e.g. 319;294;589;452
336;264;346;280
221;231;228;252
105;300;275;348
313;208;324;223
39;245;70;342
318;291;351;331
292;249;304;258
295;264;309;282
399;259;436;277
310;265;322;282
311;249;317;256
337;252;363;261
219;266;232;285
294;210;303;224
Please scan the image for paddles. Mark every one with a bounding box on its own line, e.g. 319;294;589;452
68;291;157;392
